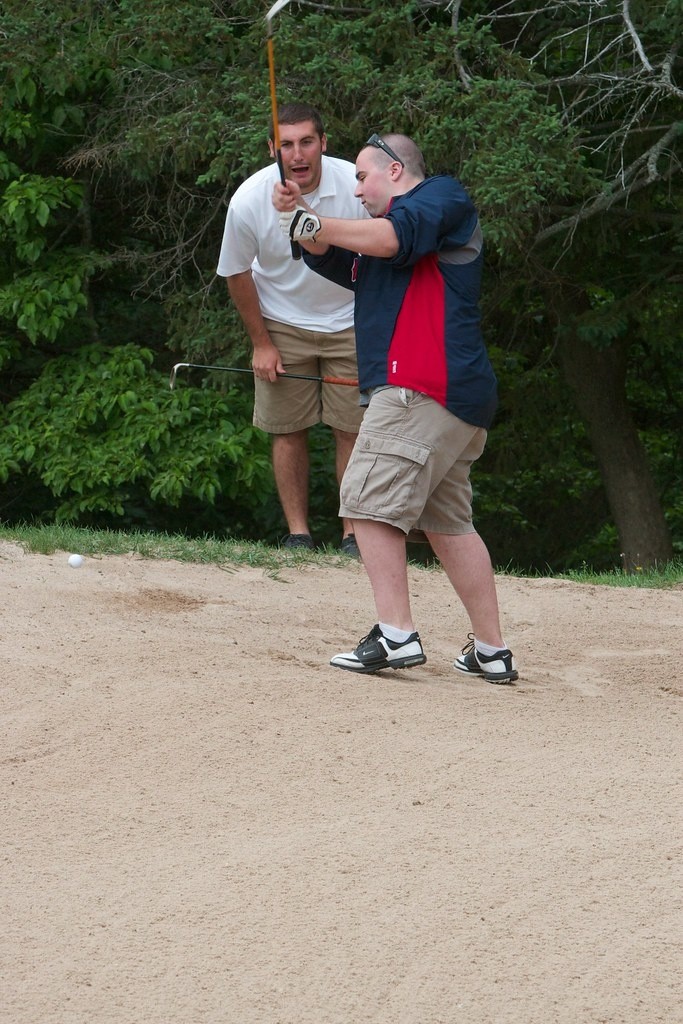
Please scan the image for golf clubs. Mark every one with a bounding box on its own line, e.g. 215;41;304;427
169;362;360;391
264;0;293;187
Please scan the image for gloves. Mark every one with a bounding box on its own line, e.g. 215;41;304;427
277;204;321;244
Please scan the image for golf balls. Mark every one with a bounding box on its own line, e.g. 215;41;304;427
67;553;83;569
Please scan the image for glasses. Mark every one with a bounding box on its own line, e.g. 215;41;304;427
365;133;405;170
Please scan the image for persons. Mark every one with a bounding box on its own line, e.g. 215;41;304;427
272;133;519;684
216;103;376;559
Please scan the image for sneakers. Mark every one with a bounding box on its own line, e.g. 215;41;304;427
338;533;362;560
329;623;428;674
452;638;519;685
284;534;315;552
